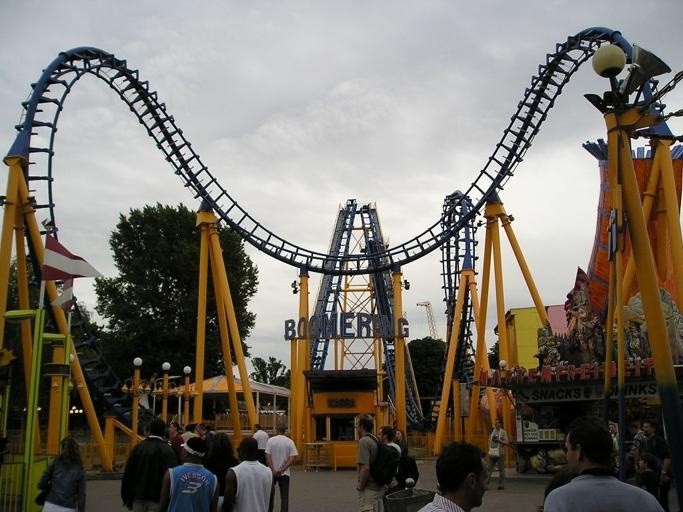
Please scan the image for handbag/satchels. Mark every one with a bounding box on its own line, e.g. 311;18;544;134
488;447;500;459
35;480;51;506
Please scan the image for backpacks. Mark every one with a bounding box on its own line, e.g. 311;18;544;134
362;433;419;488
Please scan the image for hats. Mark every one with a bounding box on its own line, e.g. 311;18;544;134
180;437;206;457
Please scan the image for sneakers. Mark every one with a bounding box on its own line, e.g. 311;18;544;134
496;486;504;490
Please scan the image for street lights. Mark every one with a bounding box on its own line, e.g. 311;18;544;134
592;44;627;481
121;357;192;454
499;360;512;468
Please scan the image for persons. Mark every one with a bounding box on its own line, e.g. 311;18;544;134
542;414;683;512
484;417;510;491
118;417;299;512
355;412;409;512
416;440;490;511
36;435;86;512
